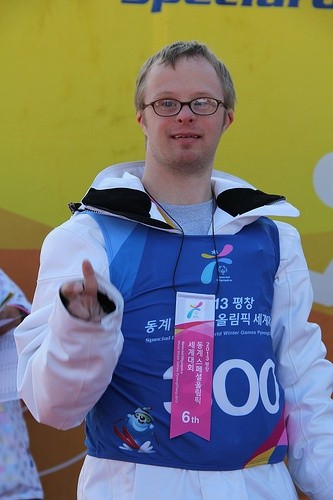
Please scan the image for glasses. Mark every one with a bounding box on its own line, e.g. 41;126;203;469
140;98;227;117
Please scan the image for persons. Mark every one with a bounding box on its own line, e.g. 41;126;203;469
13;38;333;500
1;265;45;499
